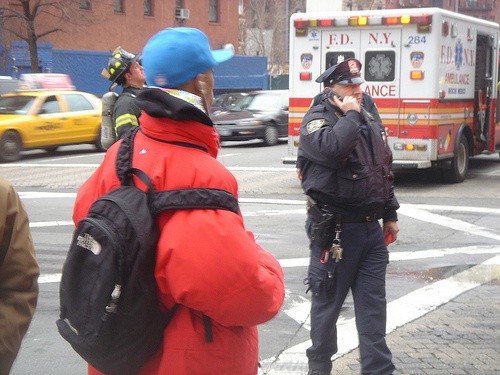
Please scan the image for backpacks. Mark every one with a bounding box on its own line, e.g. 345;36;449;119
55;127;238;375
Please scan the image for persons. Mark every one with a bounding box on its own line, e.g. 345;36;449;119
0;175;41;375
297;59;399;375
73;26;286;375
108;46;148;143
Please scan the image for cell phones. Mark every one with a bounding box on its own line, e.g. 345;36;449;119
326;90;344;103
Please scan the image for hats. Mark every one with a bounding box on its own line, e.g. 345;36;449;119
106;48;142;93
315;57;367;87
138;25;234;90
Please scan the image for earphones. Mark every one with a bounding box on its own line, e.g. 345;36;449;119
199;80;207;93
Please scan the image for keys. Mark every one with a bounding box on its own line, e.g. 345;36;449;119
329;229;343;264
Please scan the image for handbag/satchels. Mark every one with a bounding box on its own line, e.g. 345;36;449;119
304;204;337;251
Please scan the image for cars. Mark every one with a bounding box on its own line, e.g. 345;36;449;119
211;90;288;144
0;89;106;162
207;92;249;122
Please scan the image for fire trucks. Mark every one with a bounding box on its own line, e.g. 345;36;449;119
283;4;499;182
